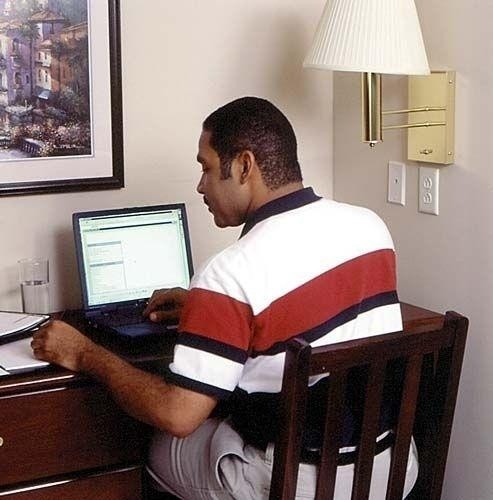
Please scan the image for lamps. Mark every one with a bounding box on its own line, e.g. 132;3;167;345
302;0;458;167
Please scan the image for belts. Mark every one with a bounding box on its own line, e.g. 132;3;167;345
249;433;394;465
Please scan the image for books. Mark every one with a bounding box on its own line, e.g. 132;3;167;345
0;336;51;379
0;310;49;338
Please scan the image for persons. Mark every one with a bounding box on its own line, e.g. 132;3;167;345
29;96;424;500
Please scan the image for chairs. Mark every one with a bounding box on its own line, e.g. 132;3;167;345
265;313;466;500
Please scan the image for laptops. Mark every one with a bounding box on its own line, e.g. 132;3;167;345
72;202;196;345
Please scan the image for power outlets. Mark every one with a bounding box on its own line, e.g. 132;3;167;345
417;167;442;216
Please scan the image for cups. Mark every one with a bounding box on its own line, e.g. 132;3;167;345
16;256;50;315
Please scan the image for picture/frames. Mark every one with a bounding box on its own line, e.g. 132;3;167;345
0;0;123;199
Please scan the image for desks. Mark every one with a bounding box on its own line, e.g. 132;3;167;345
0;304;464;500
